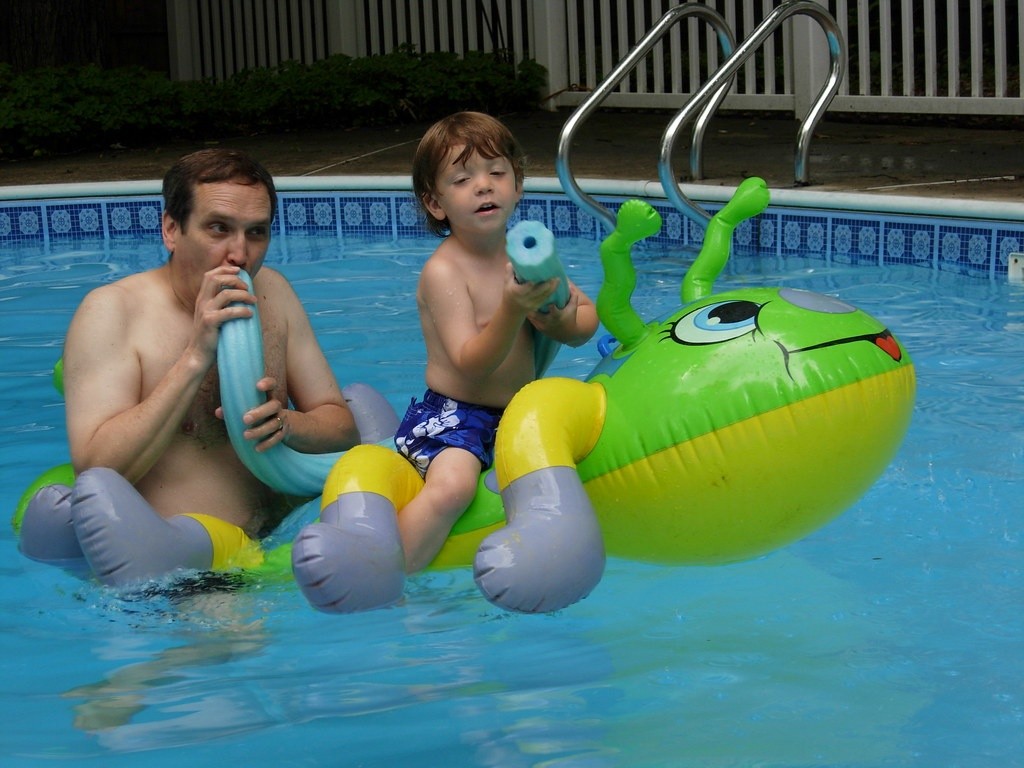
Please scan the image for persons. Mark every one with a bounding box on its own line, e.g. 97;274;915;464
389;113;600;575
64;149;362;536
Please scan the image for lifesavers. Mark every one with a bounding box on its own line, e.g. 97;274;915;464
214;219;571;499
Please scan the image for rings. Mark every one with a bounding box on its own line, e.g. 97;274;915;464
275;416;283;429
